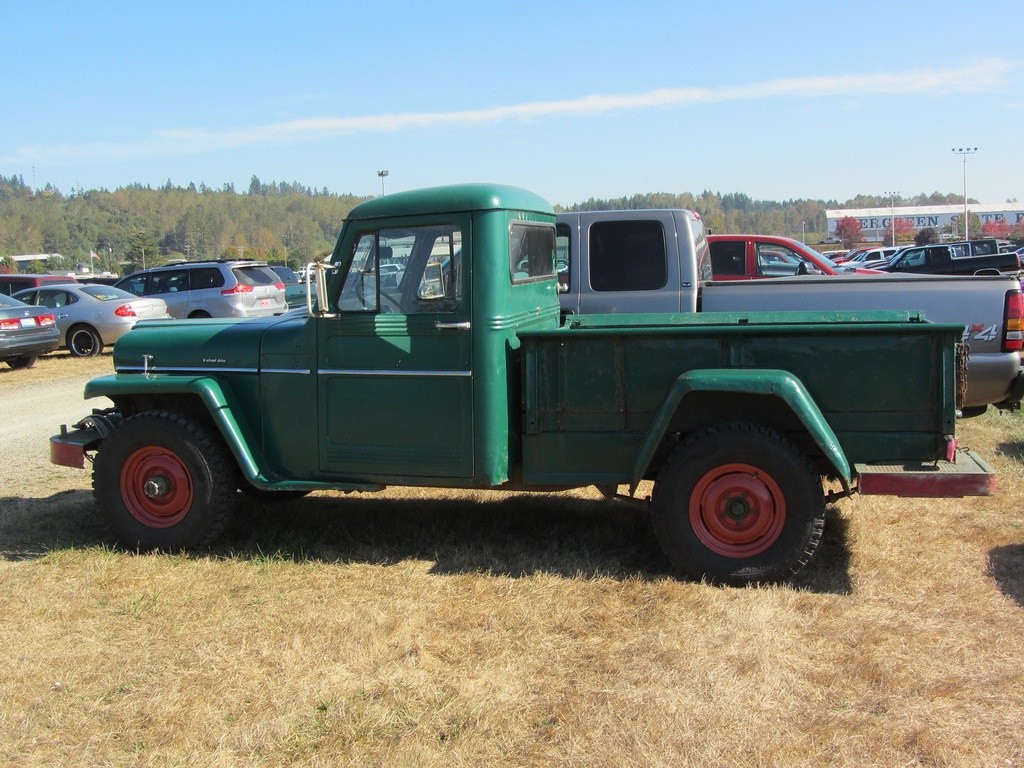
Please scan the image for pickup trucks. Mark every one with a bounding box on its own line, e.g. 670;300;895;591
439;208;1023;420
49;184;999;587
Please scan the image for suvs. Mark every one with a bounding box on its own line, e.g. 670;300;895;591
111;257;290;320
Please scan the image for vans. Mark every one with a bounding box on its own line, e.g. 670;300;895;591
0;274;78;296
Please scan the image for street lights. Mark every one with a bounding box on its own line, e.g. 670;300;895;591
376;170;388;196
884;191;900;247
952;147;978;241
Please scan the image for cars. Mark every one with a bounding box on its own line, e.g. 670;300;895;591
10;284;174;358
269;265;443;310
760;238;1024;276
0;293;60;370
554;234;887;295
